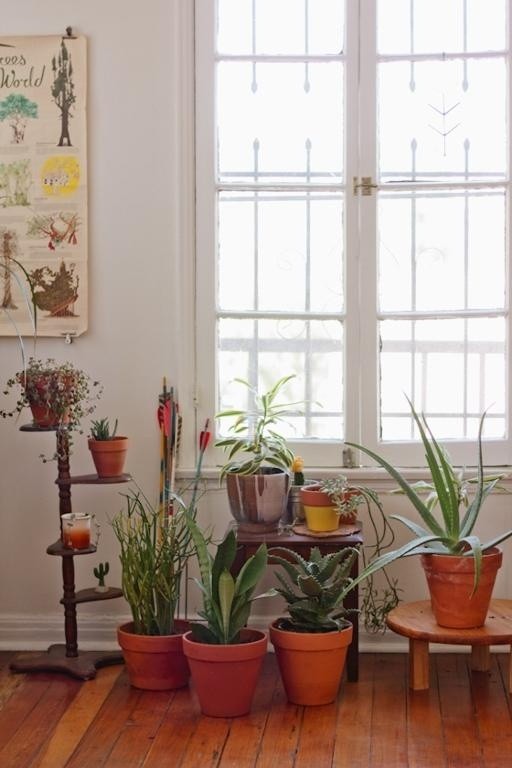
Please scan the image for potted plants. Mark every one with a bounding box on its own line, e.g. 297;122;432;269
264;543;364;706
172;518;279;723
89;418;126;476
306;473;367;535
330;390;508;628
340;484;403;634
287;456;317;520
106;501;219;690
94;561;113;594
1;357;104;462
61;508;103;551
213;372;324;531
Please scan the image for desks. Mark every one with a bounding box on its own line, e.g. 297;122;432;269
383;600;512;691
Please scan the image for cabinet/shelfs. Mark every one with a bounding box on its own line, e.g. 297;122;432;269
7;424;127;679
